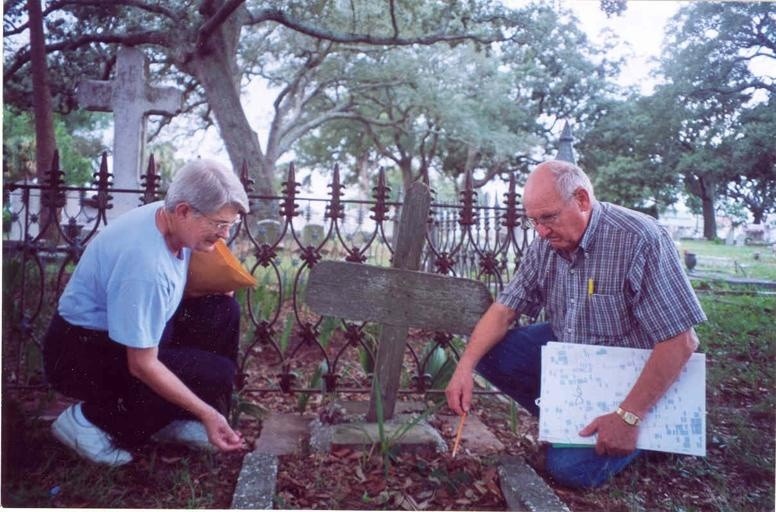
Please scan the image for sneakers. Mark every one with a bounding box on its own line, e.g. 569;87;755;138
49;405;134;467
154;419;220;450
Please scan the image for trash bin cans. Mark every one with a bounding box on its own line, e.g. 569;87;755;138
257;219;281;244
304;224;324;246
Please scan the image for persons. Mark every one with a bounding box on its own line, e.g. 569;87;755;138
42;160;250;468
443;159;709;487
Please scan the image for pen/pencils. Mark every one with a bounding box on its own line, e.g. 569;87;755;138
452;411;468;458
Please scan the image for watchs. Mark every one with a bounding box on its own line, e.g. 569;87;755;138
616;407;641;427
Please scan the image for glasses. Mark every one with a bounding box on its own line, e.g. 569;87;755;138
523;212;561;227
182;201;243;230
